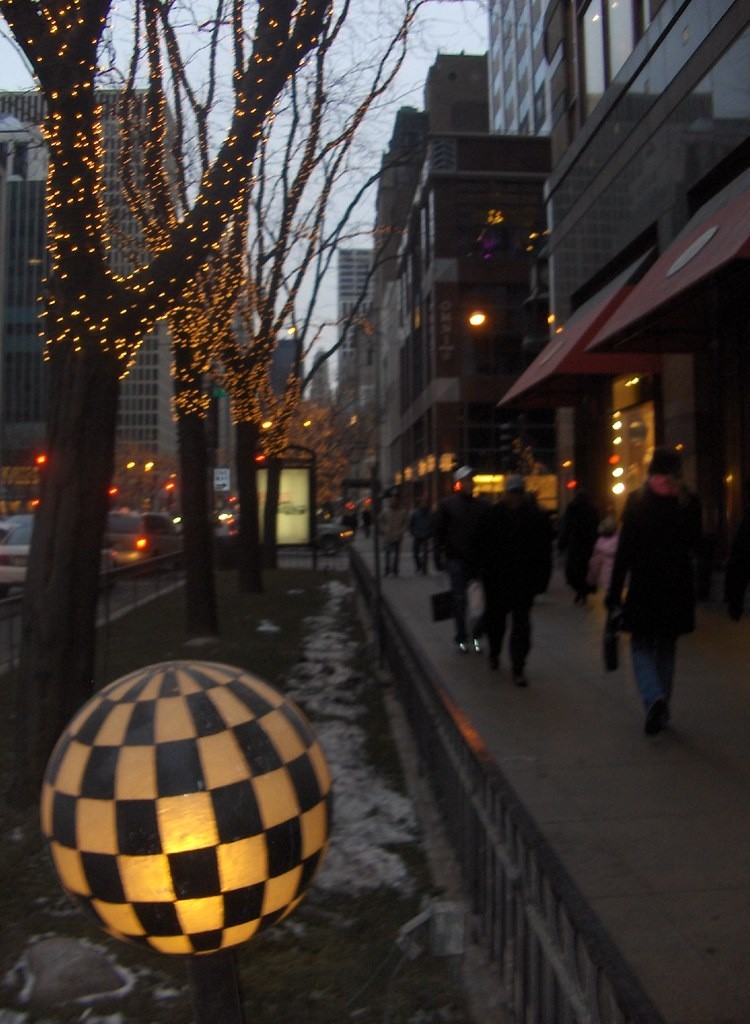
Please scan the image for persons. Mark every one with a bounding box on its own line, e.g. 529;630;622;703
553;484;604;606
320;500;371;540
587;518;632;605
407;496;436;573
474;474;555;686
375;496;410;575
608;442;706;733
429;467;490;653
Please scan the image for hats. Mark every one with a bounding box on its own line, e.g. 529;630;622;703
506;473;522;491
453;465;473;480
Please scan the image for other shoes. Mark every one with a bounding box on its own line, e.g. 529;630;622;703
472;637;482;654
508;664;526;686
642;692;673;738
487;647;500;669
458;641;466;652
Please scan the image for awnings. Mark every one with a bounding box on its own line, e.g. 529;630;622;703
493;245;661;410
584;165;749;355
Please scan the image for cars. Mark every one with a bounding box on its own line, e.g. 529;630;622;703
0;514;117;595
314;520;354;555
107;510;188;571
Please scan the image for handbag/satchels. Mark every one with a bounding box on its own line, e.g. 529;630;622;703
601;613;624;675
431;589;457;623
